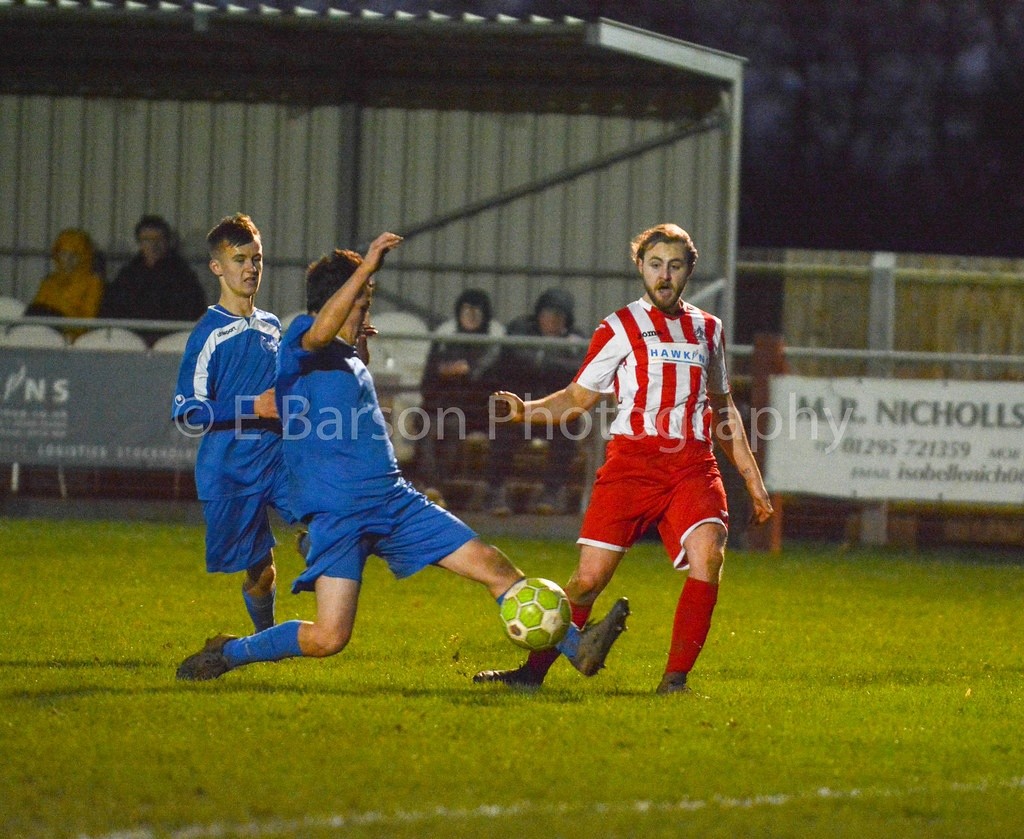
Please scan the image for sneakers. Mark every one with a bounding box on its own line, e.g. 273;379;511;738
176;632;239;681
473;663;546;688
568;597;631;676
656;672;692;695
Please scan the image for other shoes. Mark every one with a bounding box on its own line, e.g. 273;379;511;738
412;480;448;509
537;487;566;515
484;488;510;515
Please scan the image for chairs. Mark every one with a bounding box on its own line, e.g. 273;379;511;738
0;294;509;500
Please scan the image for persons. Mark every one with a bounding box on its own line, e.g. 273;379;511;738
414;289;588;514
471;224;774;694
34;213;205;347
171;212;376;635
176;230;631;680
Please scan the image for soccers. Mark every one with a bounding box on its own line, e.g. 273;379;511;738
499;575;576;652
